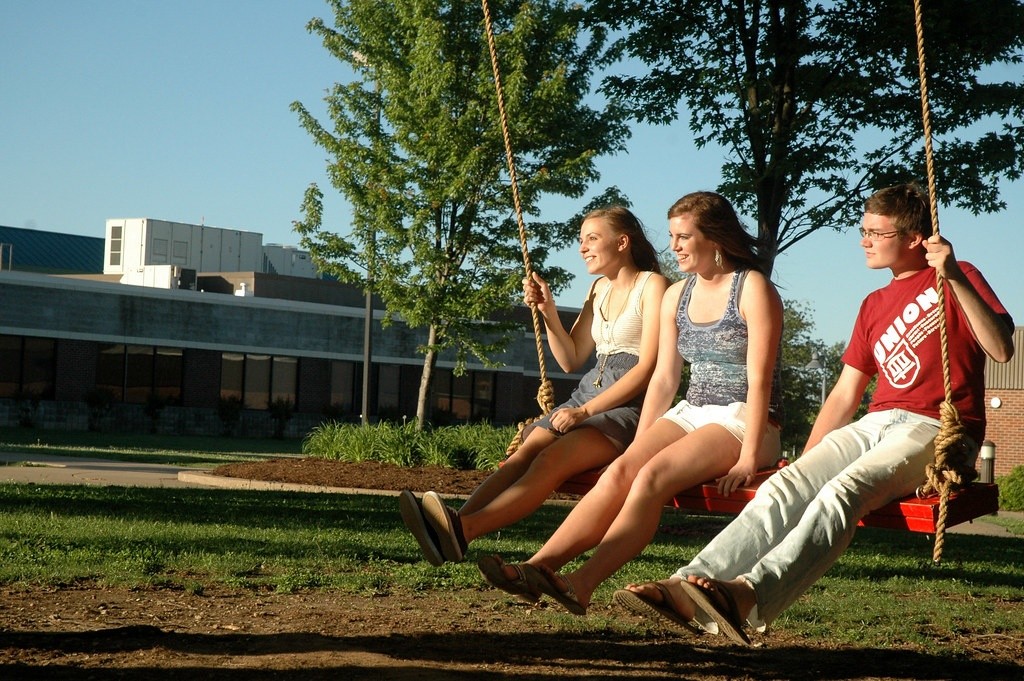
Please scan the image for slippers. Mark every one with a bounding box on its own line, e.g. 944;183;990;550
523;563;587;615
681;576;751;645
478;555;542;604
613;581;699;636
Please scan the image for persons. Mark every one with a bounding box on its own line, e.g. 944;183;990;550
473;191;784;616
611;186;1017;647
398;207;673;566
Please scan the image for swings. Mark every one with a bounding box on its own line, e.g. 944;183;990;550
479;0;999;535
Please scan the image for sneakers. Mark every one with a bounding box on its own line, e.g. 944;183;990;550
420;491;468;563
398;490;448;566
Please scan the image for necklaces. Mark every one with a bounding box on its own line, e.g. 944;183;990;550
593;269;640;388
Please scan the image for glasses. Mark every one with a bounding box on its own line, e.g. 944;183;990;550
858;226;900;241
